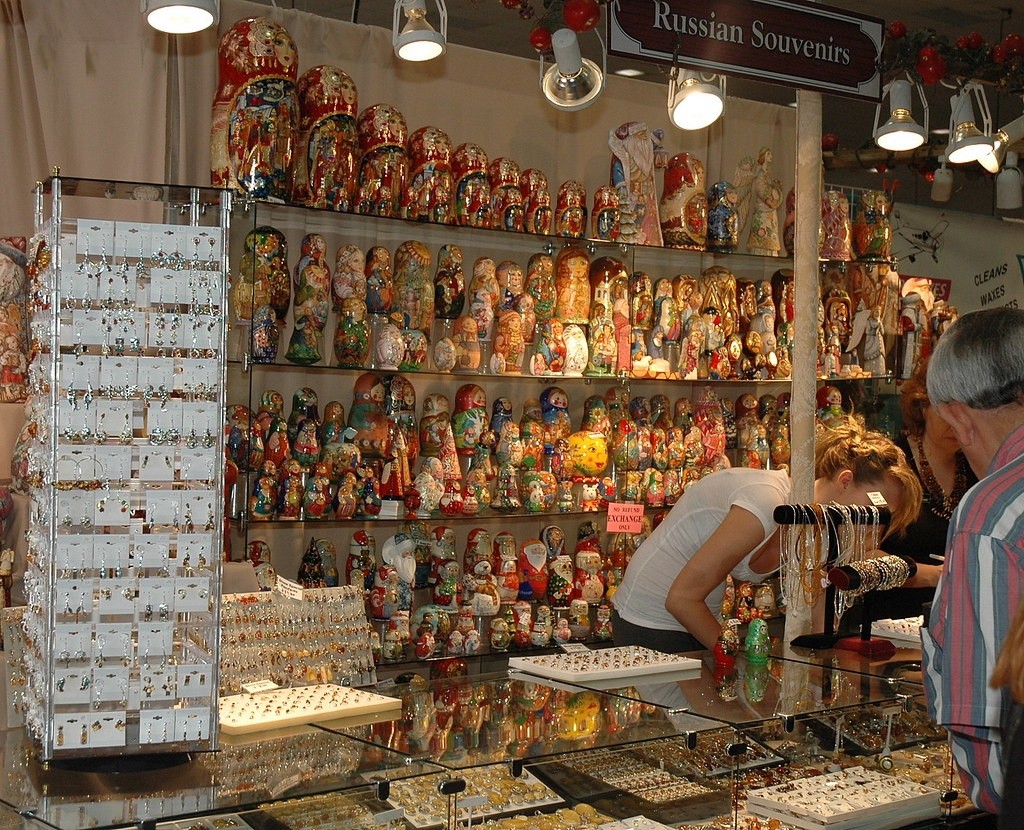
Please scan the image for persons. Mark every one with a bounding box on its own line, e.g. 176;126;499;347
609;427;922;663
838;308;1024;830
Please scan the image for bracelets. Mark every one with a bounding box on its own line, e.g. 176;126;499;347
840;557;911;595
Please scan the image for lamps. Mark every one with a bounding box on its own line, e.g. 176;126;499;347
976;114;1024;174
930;155;953;202
943;80;993;164
534;20;607;113
392;0;448;63
667;66;728;131
994;150;1024;209
140;0;222;36
872;71;930;152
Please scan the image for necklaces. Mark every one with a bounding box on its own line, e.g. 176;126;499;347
918;430;959;513
777;503;881;620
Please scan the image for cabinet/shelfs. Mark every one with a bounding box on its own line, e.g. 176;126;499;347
217;186;905;693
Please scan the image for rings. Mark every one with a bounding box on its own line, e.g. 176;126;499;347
521;647;690;673
219;685;385;723
